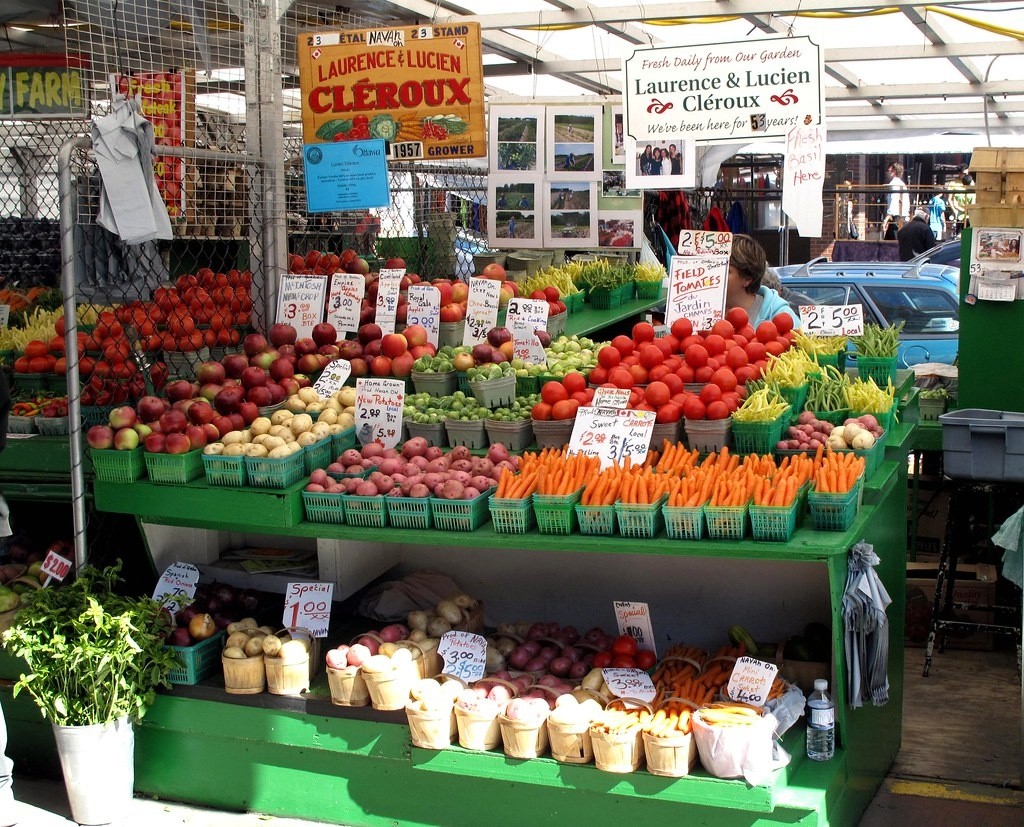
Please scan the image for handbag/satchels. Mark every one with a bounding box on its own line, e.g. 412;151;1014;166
883;219;902;232
850;218;859;238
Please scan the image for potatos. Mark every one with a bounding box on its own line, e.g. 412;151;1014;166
322;595;614;725
305;437;524;501
204;384;357;484
777;411;883;450
222;617;311;660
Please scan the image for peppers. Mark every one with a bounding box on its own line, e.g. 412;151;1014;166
7;369;146;418
918;388;947;398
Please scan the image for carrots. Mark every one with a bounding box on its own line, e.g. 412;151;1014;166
589;643;787;736
0;286;47;312
493;438;865;537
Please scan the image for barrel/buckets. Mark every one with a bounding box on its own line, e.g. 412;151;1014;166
51;713;135;826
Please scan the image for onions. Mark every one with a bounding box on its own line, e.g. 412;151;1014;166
165;583;262;645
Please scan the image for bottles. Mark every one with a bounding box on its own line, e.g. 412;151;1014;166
806;678;835;761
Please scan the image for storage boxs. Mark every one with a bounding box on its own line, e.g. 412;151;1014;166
0;283;890;780
917;399;943;420
940;409;1024;480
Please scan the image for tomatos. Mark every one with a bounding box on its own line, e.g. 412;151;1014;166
0;541;74;615
593;634;656;671
290;250;356;279
14;266;254;372
530;309;799;421
114;71;182;219
453;328;549;374
530;286;567;317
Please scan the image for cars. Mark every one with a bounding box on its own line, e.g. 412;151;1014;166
769;256;962;369
907;240;962;268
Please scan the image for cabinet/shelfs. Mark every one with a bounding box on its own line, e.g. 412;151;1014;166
99;372;917;827
0;435;86;779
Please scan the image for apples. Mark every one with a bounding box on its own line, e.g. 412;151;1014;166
322;257;521;320
87;321;435;454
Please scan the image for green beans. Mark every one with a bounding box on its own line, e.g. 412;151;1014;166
514;256;667;298
0;289;123;352
731;322;906;420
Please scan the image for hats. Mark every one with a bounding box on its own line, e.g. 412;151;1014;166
916;206;932;215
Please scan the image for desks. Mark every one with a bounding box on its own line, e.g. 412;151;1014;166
922;484;1022;676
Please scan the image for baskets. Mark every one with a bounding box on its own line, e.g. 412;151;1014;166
141;630;226;685
7;277;946;543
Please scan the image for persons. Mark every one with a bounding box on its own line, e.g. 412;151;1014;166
602;174;626;193
559;189;574;201
979;234;1019;259
833;180;854;240
897;206;936;261
519;196;529;207
508;217;516;238
497;193;507;210
928;164;976;240
881;163;910;239
640;144;683;175
600;222;634;239
725;233;802;330
568;124;575;136
562;153;576;168
761;268;817;318
